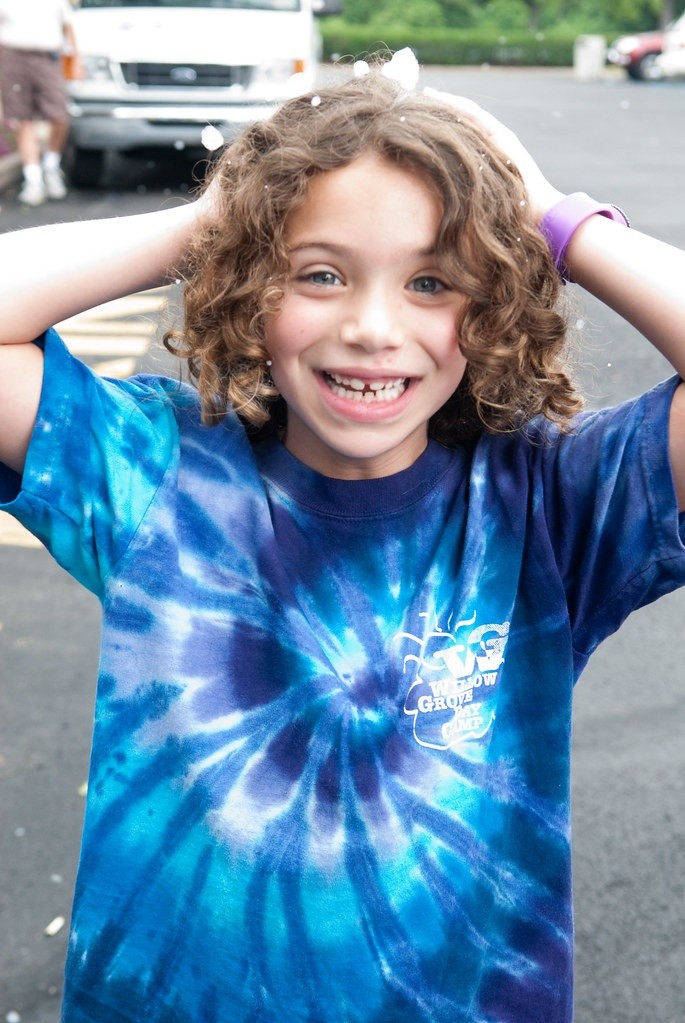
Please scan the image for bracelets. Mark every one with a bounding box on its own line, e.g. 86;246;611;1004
536;191;632;286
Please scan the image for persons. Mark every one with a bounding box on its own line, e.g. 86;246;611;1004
1;48;685;1023
0;0;86;207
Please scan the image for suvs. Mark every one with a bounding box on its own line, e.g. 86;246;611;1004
607;13;684;83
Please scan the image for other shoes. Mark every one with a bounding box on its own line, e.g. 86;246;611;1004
18;178;46;207
46;171;67;199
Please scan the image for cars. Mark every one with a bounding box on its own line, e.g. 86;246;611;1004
55;1;324;192
